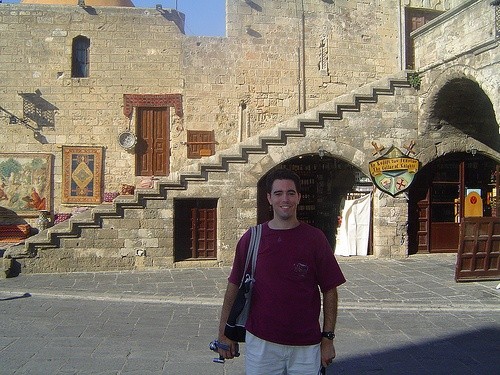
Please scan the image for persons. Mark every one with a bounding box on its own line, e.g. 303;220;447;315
215;167;347;374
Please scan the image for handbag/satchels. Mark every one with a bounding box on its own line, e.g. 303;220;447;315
224;224;262;342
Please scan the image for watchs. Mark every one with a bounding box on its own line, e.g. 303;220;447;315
321;331;336;340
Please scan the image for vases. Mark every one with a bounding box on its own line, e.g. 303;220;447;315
36;210;48;233
